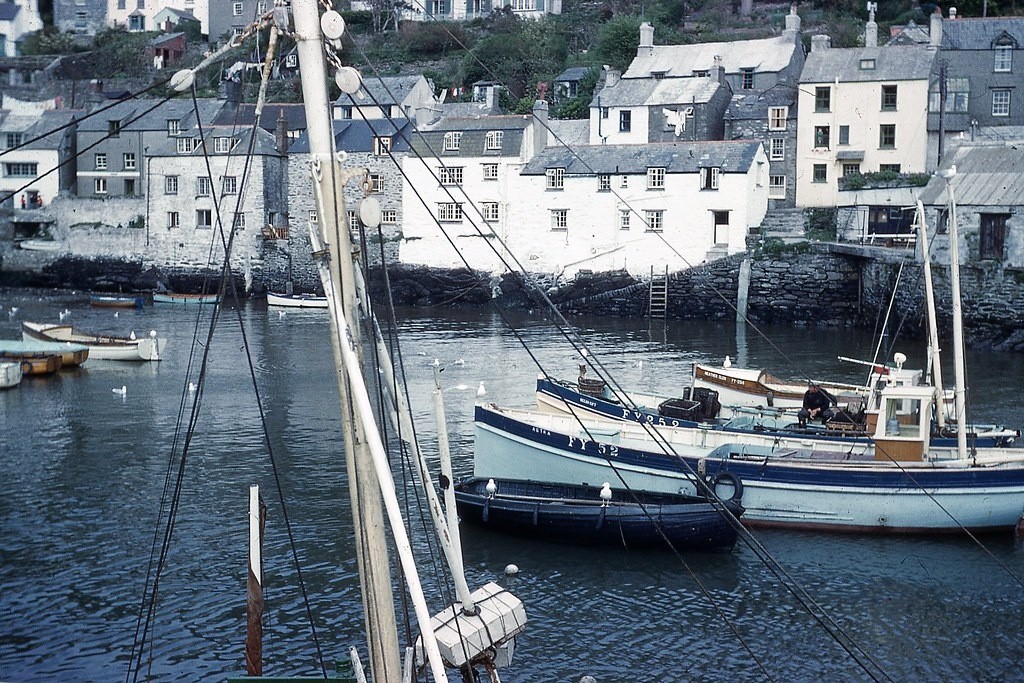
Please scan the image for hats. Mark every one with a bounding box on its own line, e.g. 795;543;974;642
808;380;820;386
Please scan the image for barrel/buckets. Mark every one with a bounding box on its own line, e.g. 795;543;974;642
578;376;605;398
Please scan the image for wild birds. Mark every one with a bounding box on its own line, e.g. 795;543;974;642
894;353;907;371
416;351;612;576
722;356;732;371
9;307;198;394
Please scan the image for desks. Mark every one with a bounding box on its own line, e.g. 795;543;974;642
827;421;866;439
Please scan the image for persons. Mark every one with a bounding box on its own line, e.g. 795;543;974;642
797;381;832;428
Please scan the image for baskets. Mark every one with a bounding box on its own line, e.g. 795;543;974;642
578;375;606;397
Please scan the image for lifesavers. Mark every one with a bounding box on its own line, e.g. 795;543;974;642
707;471;745;501
20;360;32;373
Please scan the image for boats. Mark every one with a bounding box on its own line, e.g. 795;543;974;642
0;340;90;389
473;183;1023;530
153;289;221;304
21;240;62;250
22;321;167;361
450;477;744;544
267;291;361;309
89;296;148;308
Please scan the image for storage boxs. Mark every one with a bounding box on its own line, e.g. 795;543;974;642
658;387;721;422
